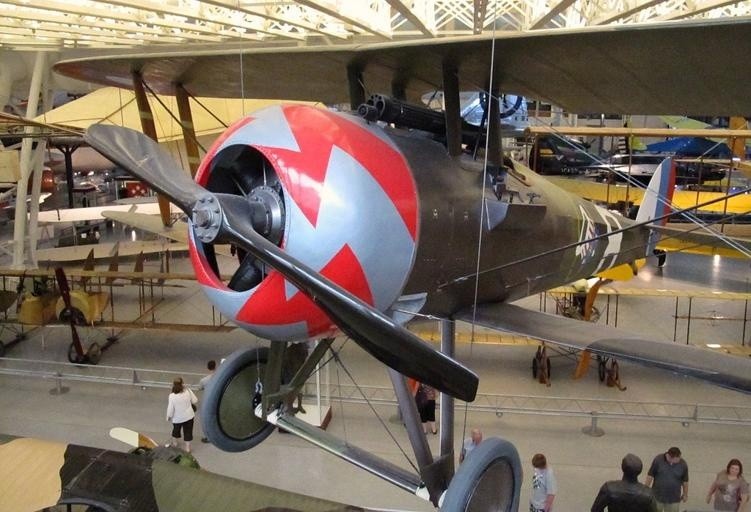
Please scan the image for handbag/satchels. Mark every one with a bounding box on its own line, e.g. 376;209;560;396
737;480;750;504
191;403;196;412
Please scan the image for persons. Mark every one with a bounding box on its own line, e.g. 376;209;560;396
644;445;690;511
529;453;558;511
591;453;659;512
167;376;199;454
415;382;439;434
198;359;218;443
458;428;483;466
706;458;750;511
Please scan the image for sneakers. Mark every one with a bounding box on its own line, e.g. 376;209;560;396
422;428;437;434
200;436;211;443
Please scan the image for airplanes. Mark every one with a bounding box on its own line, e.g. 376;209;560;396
414;281;751;392
0;270;239;365
0;427;378;512
53;17;751;512
